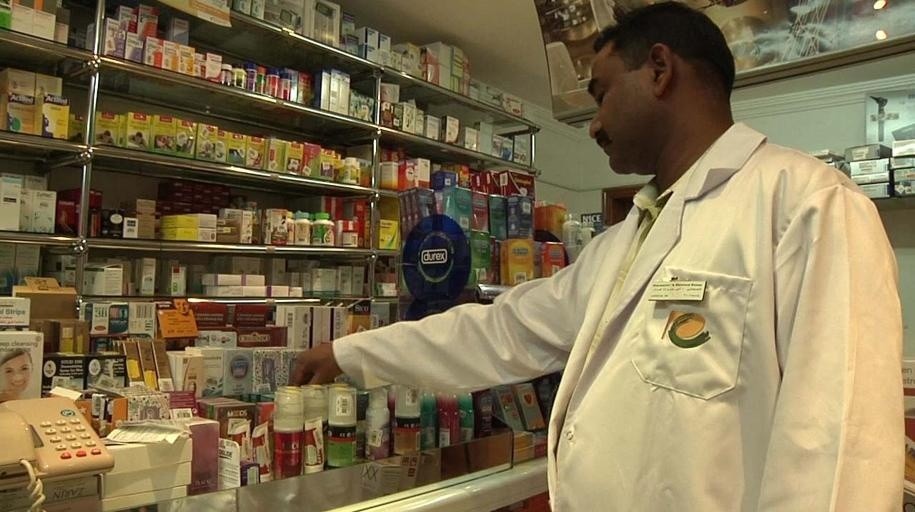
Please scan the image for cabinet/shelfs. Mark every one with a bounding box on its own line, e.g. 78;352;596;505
0;0;540;326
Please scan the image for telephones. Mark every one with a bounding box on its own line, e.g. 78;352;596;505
0;396;114;491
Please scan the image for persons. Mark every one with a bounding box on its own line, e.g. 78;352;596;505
96;130;114;145
290;1;905;512
0;348;33;403
129;132;146;149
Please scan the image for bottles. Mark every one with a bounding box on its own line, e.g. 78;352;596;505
562;218;582;265
271;375;494;482
